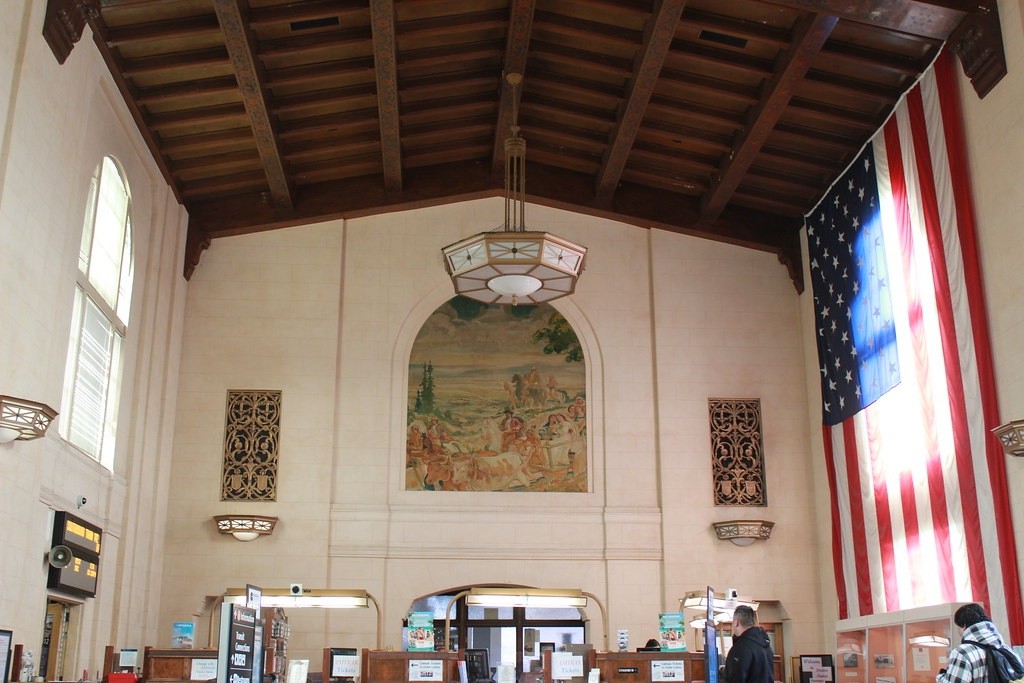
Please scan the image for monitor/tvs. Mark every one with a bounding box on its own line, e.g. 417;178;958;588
637;648;661;652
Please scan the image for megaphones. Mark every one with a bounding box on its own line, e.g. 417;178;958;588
49;545;72;568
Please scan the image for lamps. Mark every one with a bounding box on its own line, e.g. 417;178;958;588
990;419;1024;457
440;71;588;308
0;394;60;444
212;514;278;541
711;519;775;547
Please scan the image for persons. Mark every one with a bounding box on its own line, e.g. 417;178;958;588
723;605;774;683
645;639;660;647
936;603;1024;683
662;628;684;641
410;627;431;640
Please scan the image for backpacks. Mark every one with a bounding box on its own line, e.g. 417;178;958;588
962;640;1024;683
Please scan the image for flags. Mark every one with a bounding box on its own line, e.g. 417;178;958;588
801;38;1024;645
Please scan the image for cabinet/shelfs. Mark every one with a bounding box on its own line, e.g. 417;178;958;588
261;607;289;683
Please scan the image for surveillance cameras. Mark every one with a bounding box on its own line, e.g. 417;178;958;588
77;496;86;506
